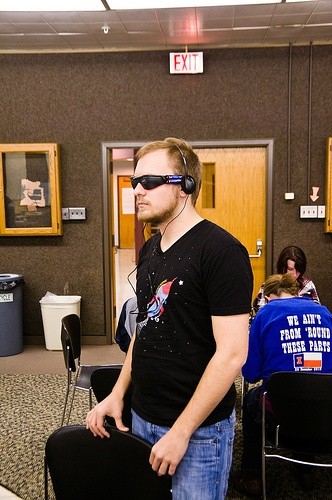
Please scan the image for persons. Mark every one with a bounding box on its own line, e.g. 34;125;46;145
233;273;332;500
254;246;320;314
85;138;253;500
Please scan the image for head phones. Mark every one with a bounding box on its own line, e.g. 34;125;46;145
172;142;196;196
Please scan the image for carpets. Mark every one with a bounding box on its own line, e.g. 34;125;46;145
0;374;263;500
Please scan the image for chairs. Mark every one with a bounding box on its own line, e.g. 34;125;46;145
44;314;332;500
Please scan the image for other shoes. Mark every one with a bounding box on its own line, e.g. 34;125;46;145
293;463;313;487
234;475;262;497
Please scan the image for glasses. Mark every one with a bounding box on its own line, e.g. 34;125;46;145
132;174;185;189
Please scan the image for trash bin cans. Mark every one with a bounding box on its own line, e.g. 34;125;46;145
40;294;82;351
0;272;25;358
